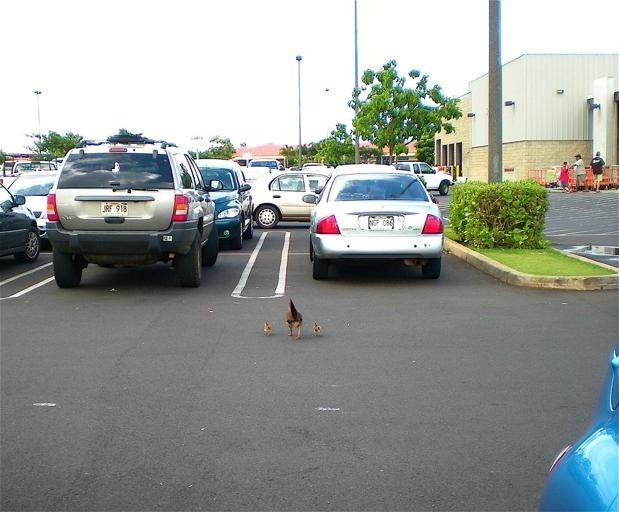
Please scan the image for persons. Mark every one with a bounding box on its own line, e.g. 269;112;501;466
569;154;589;192
590;152;605;193
558;161;570;193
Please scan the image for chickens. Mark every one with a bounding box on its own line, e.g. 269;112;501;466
312;320;322;338
285;297;303;339
263;321;271;337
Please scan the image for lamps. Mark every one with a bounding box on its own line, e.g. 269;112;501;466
504;101;514;106
591;104;600;109
467;113;475;117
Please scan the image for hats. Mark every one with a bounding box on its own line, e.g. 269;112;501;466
574;154;581;158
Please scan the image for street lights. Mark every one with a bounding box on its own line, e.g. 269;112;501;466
295;55;302;166
33;90;43;135
